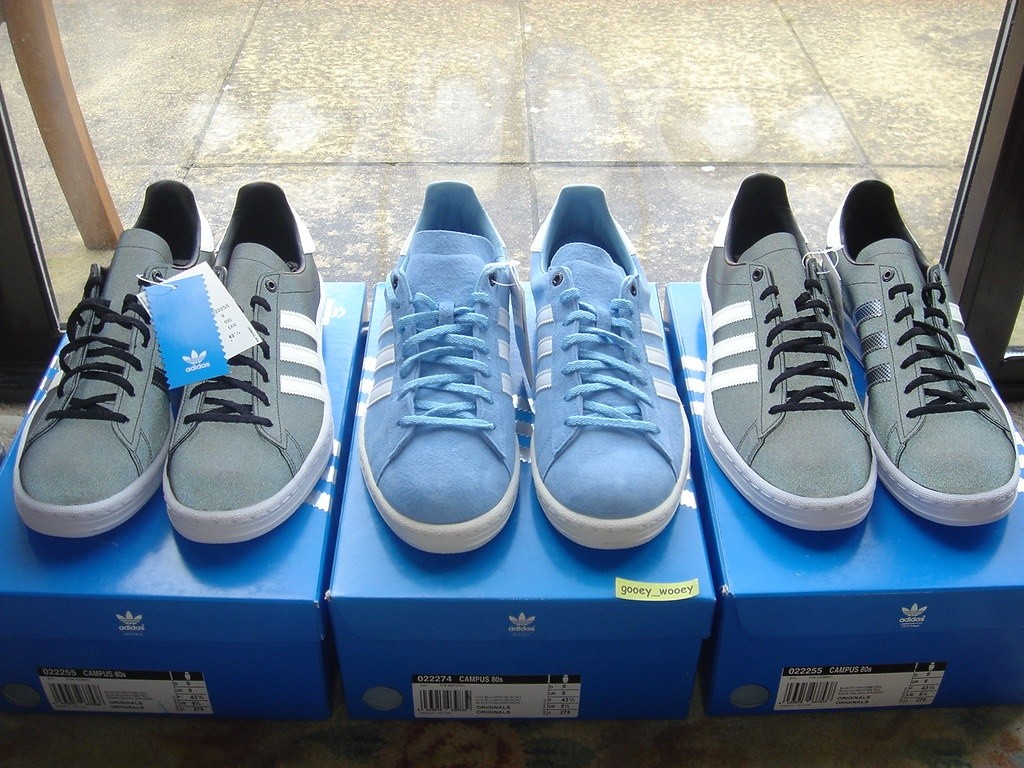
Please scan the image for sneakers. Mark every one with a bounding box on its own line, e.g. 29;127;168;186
529;182;690;550
823;180;1020;525
161;181;336;545
12;179;215;537
700;173;879;531
361;182;522;557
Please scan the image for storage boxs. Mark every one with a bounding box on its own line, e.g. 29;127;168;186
664;282;1024;720
326;281;719;720
0;278;365;718
